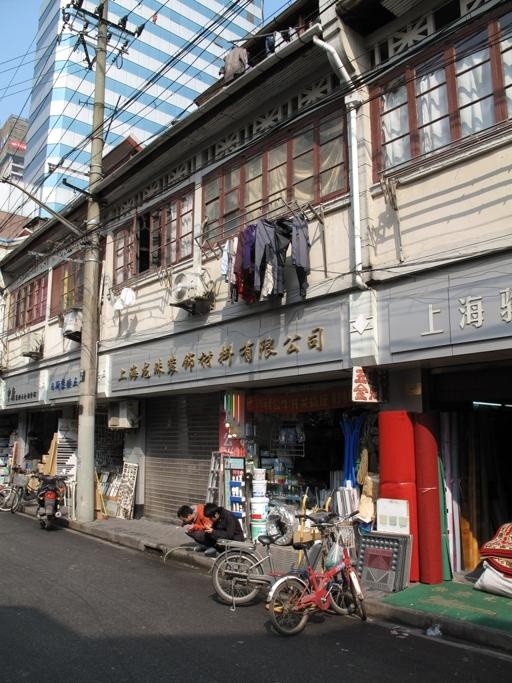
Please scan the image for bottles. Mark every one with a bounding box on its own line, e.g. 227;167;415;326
231;474;243;513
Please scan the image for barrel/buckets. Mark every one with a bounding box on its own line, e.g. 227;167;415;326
250;524;267;541
252;468;266;480
250;497;269;524
253;480;266;496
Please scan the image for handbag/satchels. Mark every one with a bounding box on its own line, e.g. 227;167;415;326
325;541;344;569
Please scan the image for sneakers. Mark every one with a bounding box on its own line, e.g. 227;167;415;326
194;544;217;555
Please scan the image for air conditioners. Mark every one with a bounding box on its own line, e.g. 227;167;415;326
108;399;139;431
21;332;42;356
62;311;83;335
170;270;209;305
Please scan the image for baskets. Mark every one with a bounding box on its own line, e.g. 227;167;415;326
328;526;355;548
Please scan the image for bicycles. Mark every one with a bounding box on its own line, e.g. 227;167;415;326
267;511;367;636
0;467;68;514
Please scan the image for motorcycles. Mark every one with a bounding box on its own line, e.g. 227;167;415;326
31;473;69;530
210;510;358;615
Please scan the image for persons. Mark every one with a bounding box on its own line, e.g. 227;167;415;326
201;503;245;558
176;504;214;552
